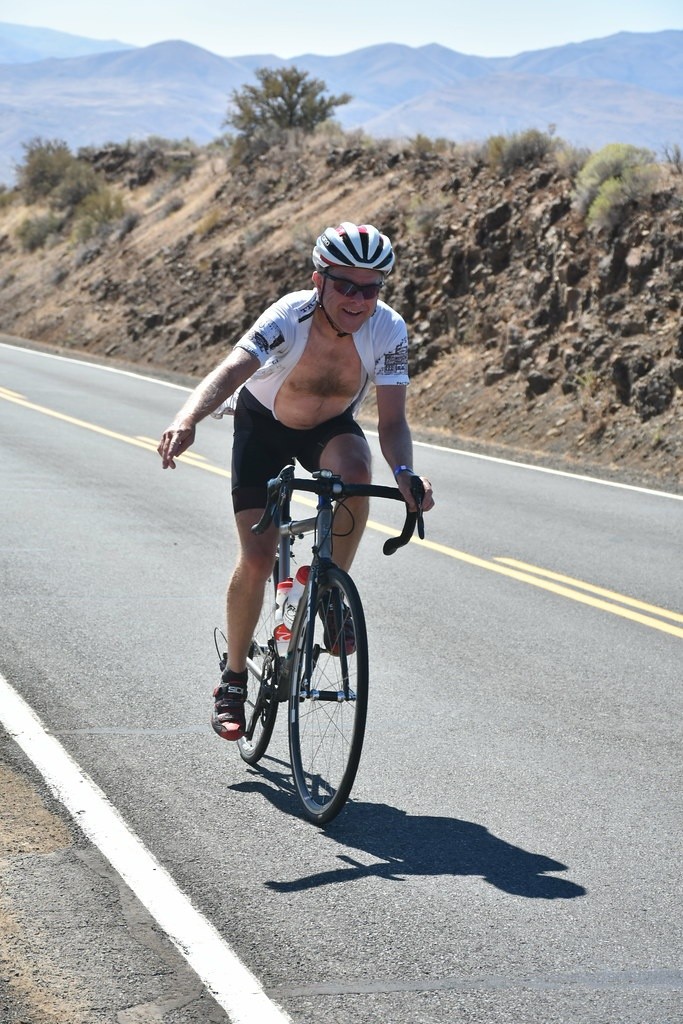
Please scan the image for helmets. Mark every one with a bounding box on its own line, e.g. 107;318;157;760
313;222;394;277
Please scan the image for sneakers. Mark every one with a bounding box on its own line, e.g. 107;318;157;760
211;680;248;741
320;592;356;656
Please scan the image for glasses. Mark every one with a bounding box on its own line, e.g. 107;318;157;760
321;272;382;300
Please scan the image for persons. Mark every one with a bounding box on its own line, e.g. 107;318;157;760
157;221;435;742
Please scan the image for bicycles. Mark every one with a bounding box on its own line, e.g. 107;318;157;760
213;464;425;827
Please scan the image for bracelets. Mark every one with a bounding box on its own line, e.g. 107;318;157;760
393;465;416;484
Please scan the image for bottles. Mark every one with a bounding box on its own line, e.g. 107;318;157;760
274;577;294;657
283;565;311;630
